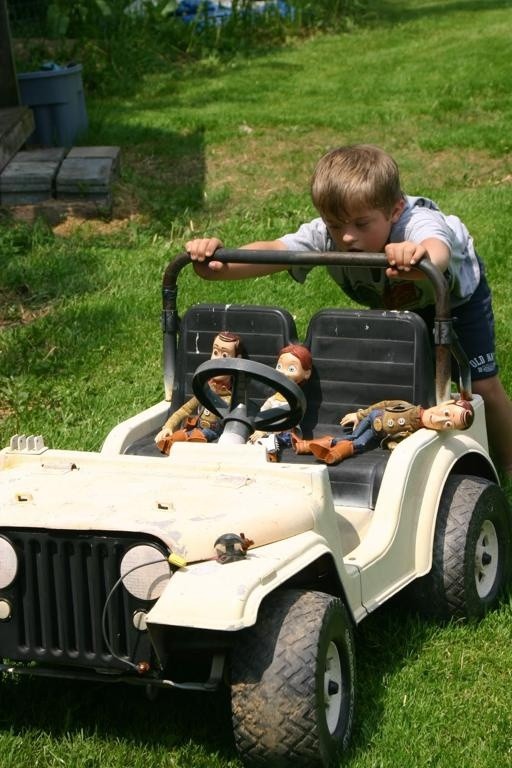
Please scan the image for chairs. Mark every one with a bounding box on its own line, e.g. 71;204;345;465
279;307;433;510
127;307;298;462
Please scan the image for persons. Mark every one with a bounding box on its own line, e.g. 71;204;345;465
154;326;246;454
185;140;511;474
288;386;476;467
247;337;312;462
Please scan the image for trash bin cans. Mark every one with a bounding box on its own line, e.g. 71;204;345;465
16;61;89;147
